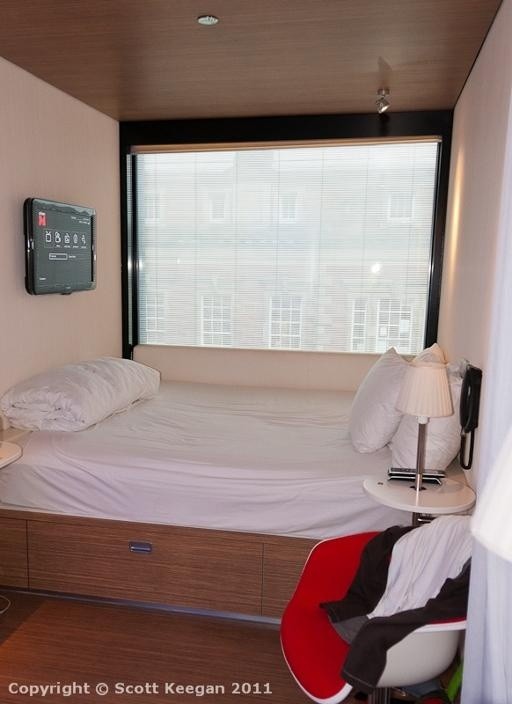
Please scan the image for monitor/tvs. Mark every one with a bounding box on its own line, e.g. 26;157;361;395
23;197;98;297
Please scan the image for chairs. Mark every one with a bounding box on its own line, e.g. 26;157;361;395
274;523;469;702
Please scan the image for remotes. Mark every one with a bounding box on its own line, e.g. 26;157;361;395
388;467;445;477
389;475;440;484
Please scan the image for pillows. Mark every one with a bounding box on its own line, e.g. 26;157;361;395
347;344;469;477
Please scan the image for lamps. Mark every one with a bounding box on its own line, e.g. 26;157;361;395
393;362;456;493
375;88;391;114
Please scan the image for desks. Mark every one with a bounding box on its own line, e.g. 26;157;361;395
359;476;479;529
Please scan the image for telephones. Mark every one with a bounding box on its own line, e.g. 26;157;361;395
460;367;482;433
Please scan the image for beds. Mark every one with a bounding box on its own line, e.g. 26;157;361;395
0;378;465;628
